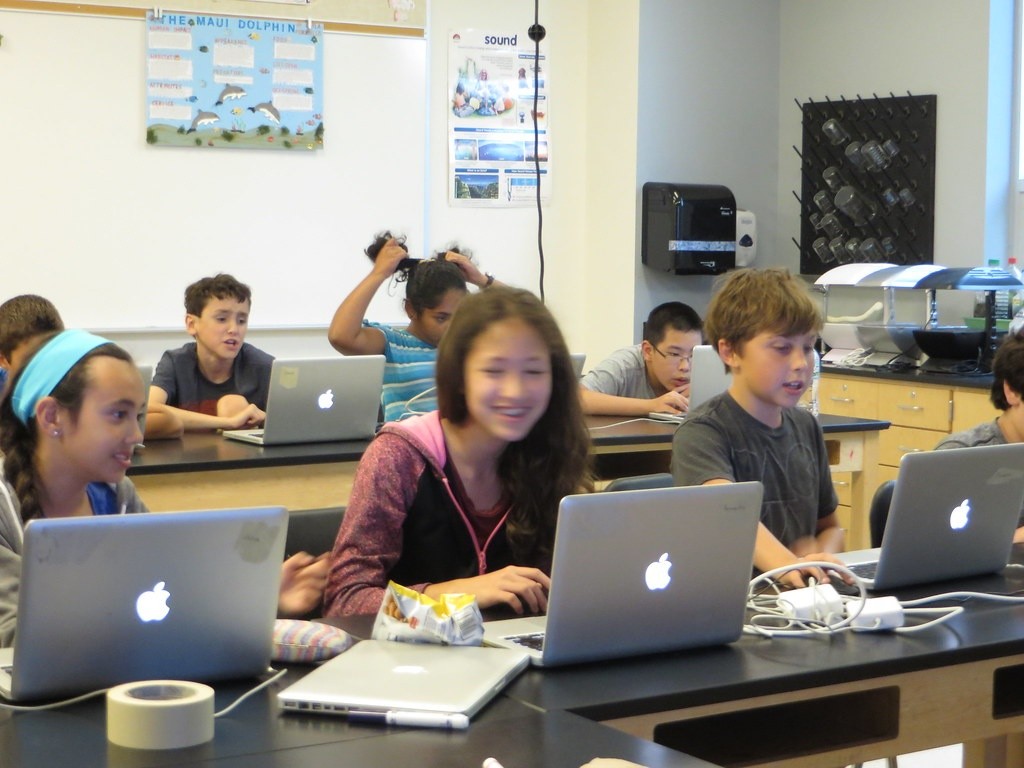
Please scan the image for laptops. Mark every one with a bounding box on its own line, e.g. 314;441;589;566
830;442;1024;592
278;638;531;721
222;355;387;445
481;481;764;667
648;345;733;423
0;506;291;702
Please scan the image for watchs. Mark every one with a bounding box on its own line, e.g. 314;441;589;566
484;272;494;287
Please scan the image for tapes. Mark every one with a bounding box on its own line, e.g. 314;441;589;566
105;680;216;751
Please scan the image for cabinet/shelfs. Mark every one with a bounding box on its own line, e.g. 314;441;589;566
796;374;1005;552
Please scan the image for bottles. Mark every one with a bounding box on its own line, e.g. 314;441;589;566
973;258;1022;318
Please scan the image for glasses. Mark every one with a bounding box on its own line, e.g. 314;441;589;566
651;344;693;363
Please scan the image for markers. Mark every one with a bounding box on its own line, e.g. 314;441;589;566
348;709;469;730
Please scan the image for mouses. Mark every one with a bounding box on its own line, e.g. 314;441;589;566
803;573;861;597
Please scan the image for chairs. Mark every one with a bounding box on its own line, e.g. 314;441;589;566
279;472;902;622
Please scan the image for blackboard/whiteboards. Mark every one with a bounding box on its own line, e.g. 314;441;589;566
0;8;429;334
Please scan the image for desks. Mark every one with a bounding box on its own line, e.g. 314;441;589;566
0;541;1024;768
125;411;891;555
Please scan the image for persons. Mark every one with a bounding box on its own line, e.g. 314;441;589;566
328;231;520;443
322;287;597;617
0;294;74;417
935;322;1024;544
670;268;855;587
146;272;276;428
578;301;703;413
0;330;330;652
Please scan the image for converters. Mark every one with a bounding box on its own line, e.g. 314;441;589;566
846;596;904;632
779;583;844;624
